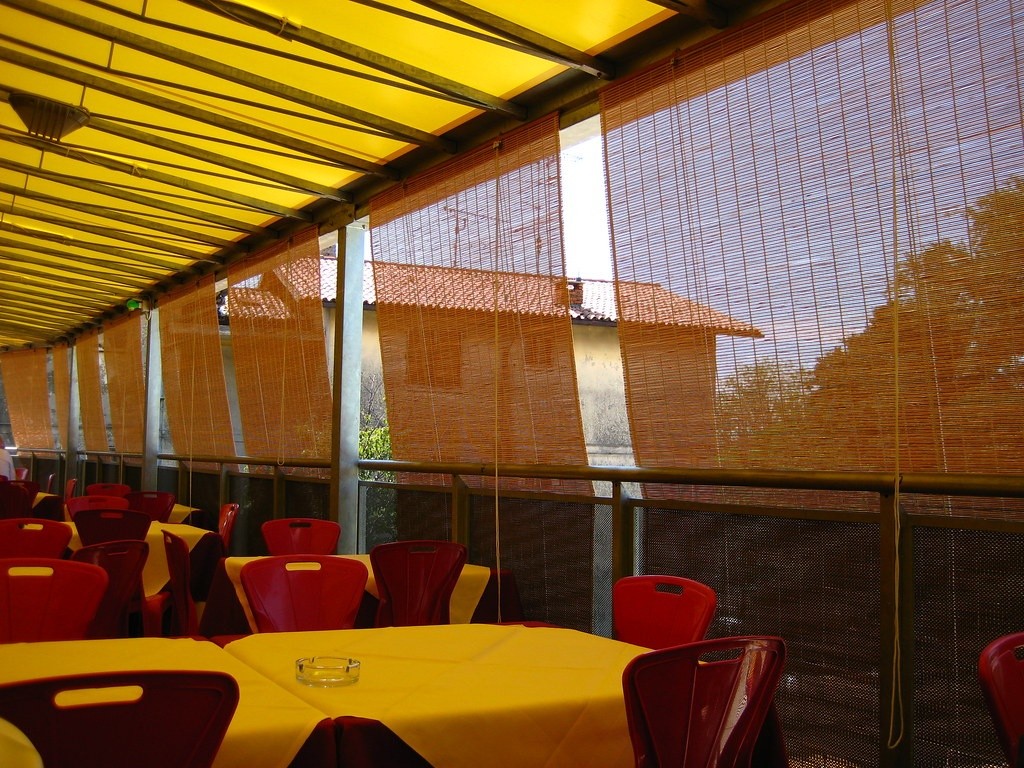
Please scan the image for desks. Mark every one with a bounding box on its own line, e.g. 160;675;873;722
213;622;705;768
207;554;490;636
167;503;202;522
31;492;59;510
26;522;217;595
0;635;333;768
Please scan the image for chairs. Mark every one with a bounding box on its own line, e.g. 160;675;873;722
979;630;1024;768
0;467;787;768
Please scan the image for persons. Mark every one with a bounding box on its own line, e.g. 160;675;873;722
0;435;16;480
